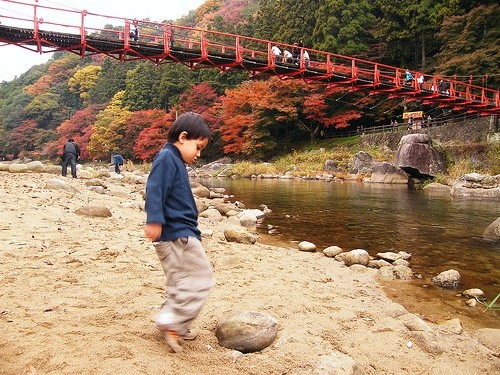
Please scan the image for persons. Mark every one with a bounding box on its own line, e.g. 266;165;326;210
405;68;451;95
146;111;217;353
61;138;81;178
130;17;140;42
170;23;175;48
114;153;123;174
271;43;311;67
358;115;432;135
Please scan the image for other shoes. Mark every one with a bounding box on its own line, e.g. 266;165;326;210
73;175;77;178
180;326;197;340
155;324;183;353
